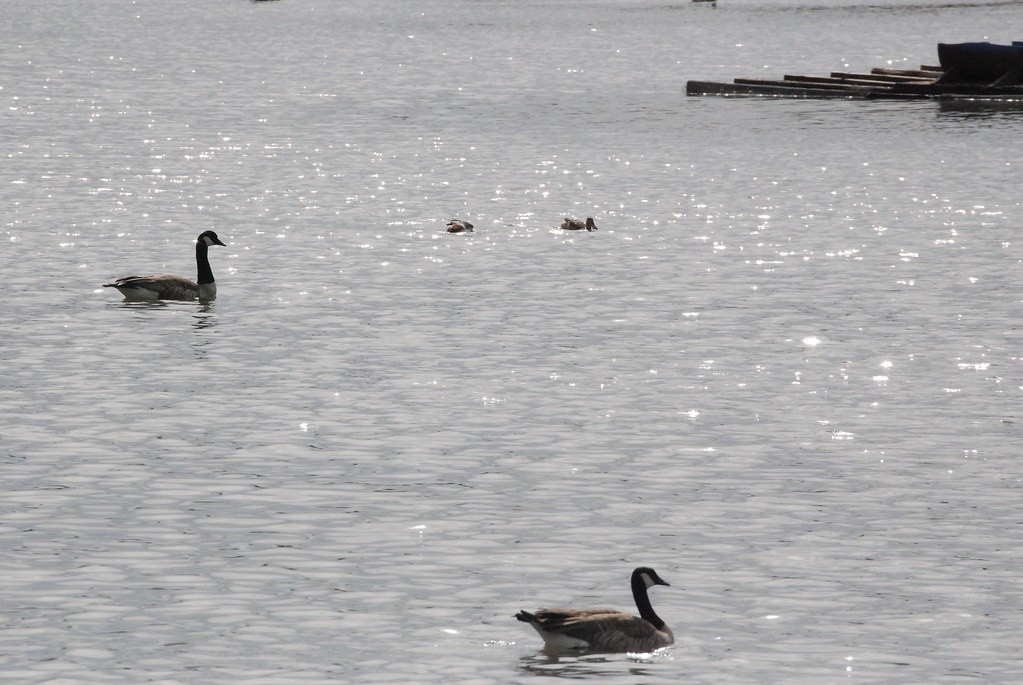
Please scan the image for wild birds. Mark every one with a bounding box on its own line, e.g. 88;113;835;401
102;230;228;303
561;217;599;232
512;567;676;655
446;218;474;235
